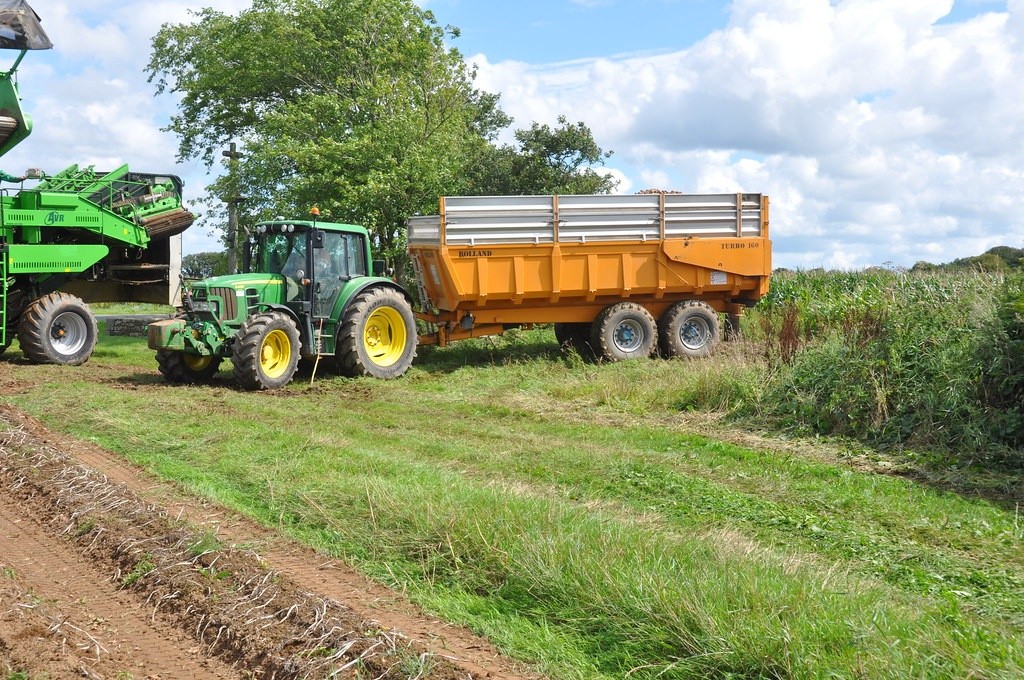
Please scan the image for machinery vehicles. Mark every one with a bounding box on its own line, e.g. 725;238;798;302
0;1;196;365
146;192;772;392
